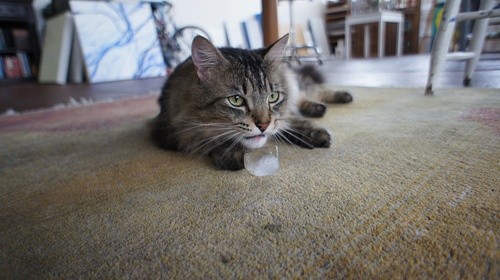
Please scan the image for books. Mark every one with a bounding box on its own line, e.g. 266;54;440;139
0;11;82;86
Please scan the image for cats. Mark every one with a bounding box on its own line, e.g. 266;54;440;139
153;32;353;171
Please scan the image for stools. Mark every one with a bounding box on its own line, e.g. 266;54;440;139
344;10;404;58
277;1;322;65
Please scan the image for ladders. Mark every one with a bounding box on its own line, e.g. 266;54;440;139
424;0;500;96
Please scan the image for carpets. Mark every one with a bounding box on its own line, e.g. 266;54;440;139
0;81;500;280
